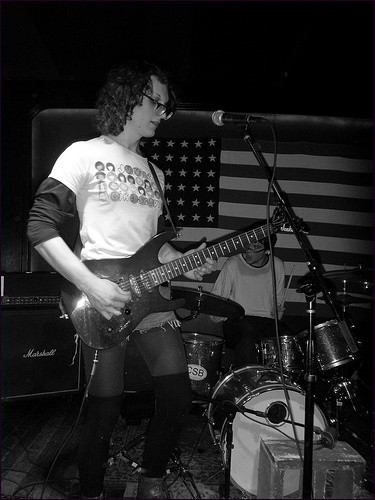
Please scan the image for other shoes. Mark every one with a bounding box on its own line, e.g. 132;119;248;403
136;475;172;500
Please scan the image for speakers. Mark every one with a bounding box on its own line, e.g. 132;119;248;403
0;304;85;400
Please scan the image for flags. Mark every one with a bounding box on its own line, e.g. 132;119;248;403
138;136;372;321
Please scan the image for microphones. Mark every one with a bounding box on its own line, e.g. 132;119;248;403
322;427;339;447
212;110;269;126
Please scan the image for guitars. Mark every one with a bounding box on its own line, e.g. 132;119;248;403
59;200;295;351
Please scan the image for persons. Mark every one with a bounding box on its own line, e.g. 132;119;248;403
27;60;217;500
210;222;315;383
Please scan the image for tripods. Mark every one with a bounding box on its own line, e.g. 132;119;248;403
108;435;203;499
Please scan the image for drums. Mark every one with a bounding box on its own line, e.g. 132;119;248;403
255;335;305;375
202;364;331;499
181;332;225;398
297;318;364;371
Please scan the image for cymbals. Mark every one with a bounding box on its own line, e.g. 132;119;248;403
321;268;370;283
173;286;245;319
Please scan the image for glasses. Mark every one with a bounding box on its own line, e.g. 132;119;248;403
143;92;173;120
243;246;266;255
